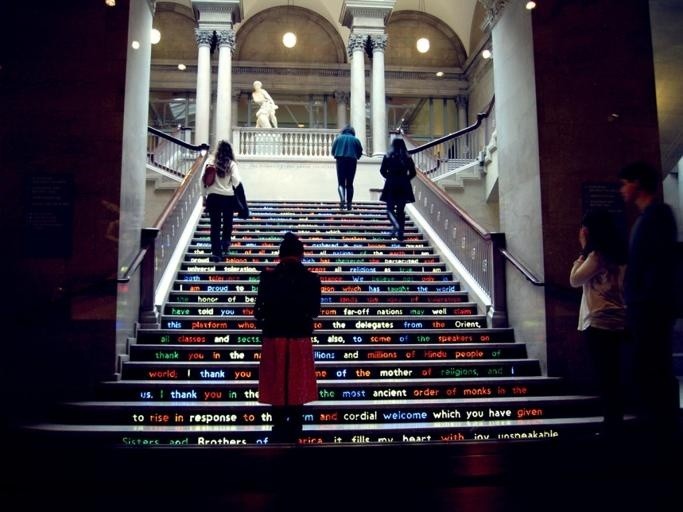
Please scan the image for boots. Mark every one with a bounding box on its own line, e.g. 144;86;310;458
338;185;345;209
346;185;353;210
386;211;407;240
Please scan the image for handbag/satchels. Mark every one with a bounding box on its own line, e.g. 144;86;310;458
202;164;216;188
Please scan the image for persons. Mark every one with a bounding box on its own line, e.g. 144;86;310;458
332;125;364;210
611;154;682;473
250;80;280;130
255;99;279;128
198;139;241;261
253;231;325;448
379;137;417;240
565;211;628;444
